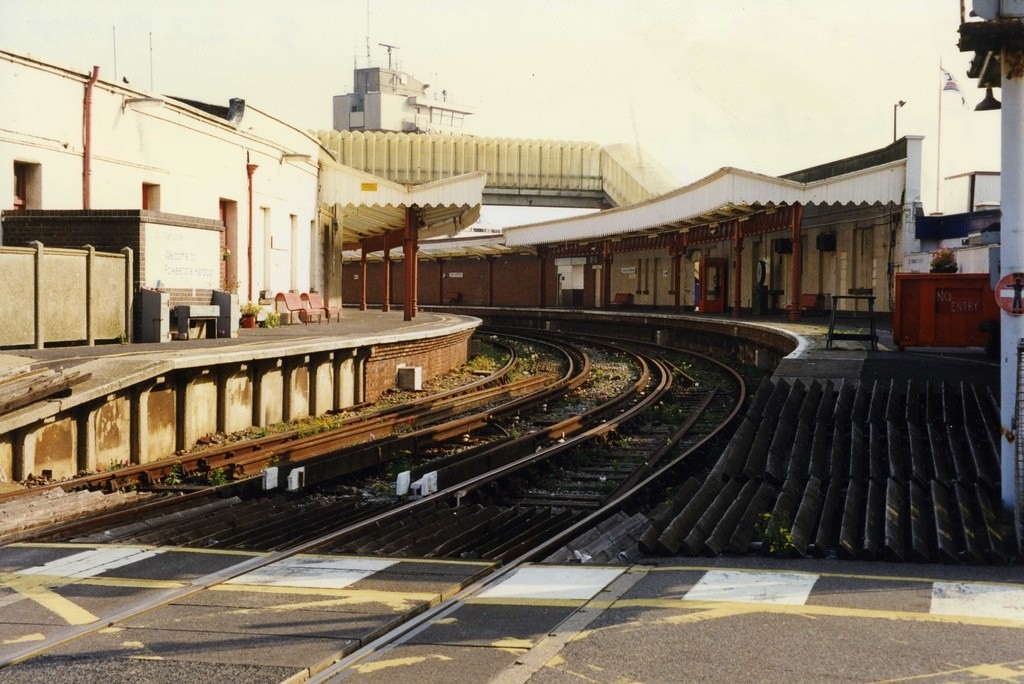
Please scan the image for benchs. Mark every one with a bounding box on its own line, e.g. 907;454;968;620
786;294;820;322
608;293;631;304
299;292;340;323
274;292;322;326
442;292;461;302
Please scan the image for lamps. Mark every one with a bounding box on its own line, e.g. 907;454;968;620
974;83;1002;111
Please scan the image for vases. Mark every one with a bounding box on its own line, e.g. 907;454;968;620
930;265;959;274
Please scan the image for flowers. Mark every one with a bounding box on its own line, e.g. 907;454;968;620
929;248;956;269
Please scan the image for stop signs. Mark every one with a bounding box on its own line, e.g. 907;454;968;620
995;272;1024;314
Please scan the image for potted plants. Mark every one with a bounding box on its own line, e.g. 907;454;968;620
240;301;260;328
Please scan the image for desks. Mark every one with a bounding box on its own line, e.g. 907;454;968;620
825;295;880;350
